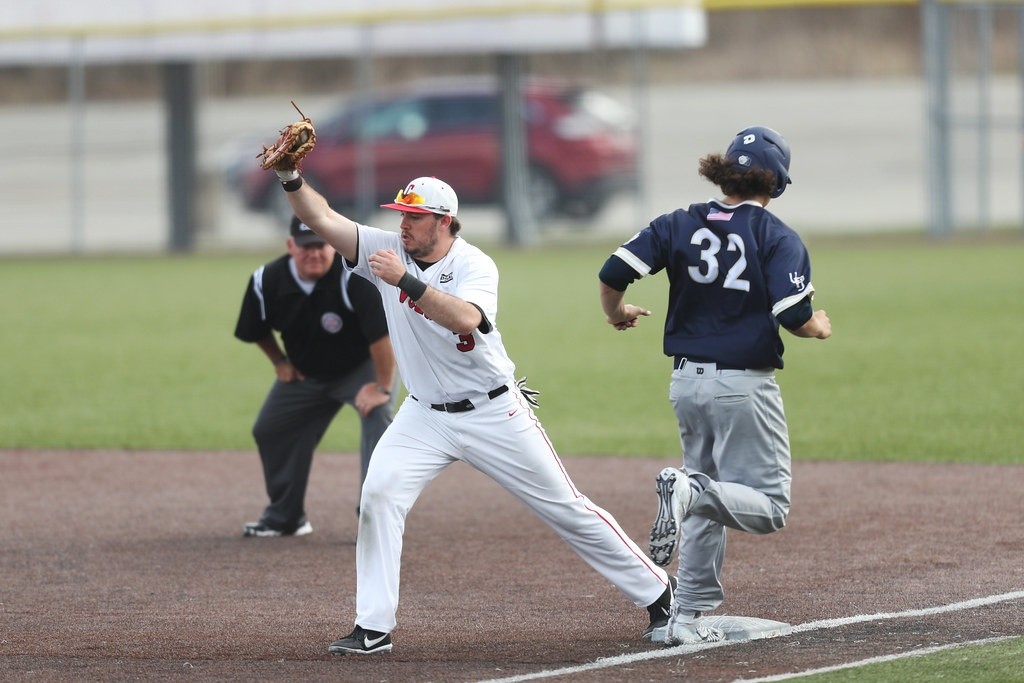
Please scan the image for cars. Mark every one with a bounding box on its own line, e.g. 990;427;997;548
234;79;641;221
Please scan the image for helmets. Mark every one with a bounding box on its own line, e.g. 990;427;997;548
725;126;792;198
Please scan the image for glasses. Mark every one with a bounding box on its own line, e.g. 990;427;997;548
395;189;451;212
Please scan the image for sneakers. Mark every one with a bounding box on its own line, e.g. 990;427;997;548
328;625;392;654
642;575;678;640
649;467;693;566
665;618;726;646
244;520;313;537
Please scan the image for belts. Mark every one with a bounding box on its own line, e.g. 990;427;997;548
674;355;745;371
412;385;508;413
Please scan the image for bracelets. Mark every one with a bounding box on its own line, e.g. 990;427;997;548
281;174;302;192
372;382;391;395
396;271;427;302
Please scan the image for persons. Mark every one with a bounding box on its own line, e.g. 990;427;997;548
234;213;396;538
265;130;680;654
597;127;833;645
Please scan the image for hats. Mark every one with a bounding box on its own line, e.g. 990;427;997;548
380;176;459;219
290;216;327;246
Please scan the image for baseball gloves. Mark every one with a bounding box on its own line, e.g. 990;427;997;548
255;118;318;176
